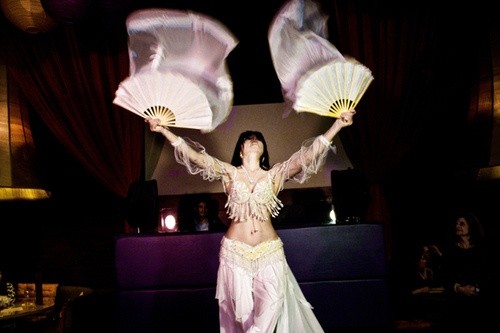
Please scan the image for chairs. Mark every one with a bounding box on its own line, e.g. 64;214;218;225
16;286;95;333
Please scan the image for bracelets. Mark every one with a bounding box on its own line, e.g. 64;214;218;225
170;137;184;147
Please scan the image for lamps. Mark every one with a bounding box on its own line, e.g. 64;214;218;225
157;207;180;233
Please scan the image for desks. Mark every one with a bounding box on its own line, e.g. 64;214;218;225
0;301;54;333
115;224;388;292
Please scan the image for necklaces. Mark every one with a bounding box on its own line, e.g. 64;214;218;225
240;165;261;182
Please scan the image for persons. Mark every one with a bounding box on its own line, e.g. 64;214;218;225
409;210;492;333
145;109;356;333
187;200;224;233
